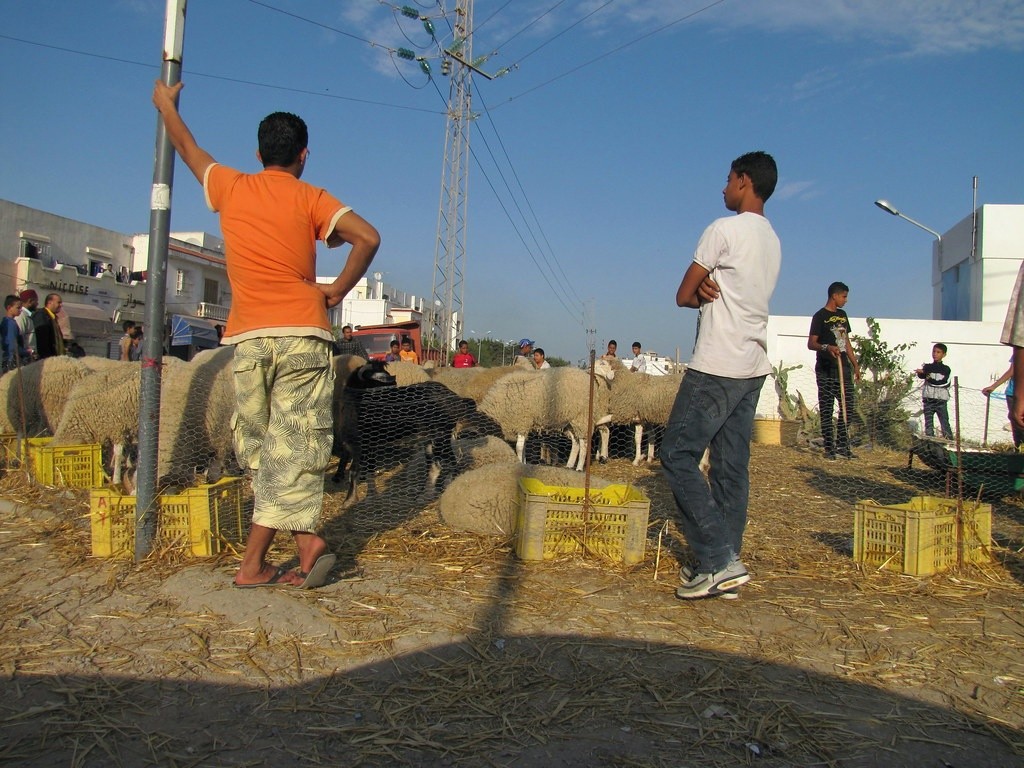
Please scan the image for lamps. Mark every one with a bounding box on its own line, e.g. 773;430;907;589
874;199;942;246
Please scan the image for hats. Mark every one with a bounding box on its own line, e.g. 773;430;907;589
518;339;535;348
19;289;38;300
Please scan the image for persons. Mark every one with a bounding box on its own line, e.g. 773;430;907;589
153;80;381;589
916;343;953;439
629;342;647;372
659;152;783;597
399;338;418;365
119;321;145;361
983;260;1024;448
533;348;551;369
32;293;66;358
451;340;479;368
807;282;860;460
601;339;617;359
104;263;114;273
14;289;38;360
0;294;32;372
333;326;368;360
386;340;400;362
512;338;535;366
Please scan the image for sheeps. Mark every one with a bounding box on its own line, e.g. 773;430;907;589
405;436;519;490
594;353;711;469
450;367;607;469
332;354;538;506
44;355;187;485
123;346;236;496
0;355;121;466
440;464;648;539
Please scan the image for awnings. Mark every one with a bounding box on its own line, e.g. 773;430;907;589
58;302;112;339
170;314;219;348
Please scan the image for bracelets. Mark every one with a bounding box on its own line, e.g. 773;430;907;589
822;344;829;351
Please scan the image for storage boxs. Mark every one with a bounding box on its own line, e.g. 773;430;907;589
853;496;992;577
90;476;245;560
514;477;651;565
20;437;104;489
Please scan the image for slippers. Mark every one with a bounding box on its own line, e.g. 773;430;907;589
295;553;337;589
236;568;295;587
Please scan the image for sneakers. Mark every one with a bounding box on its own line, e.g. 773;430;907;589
833;447;859;459
678;564;738;600
676;551;751;598
825;450;837;463
